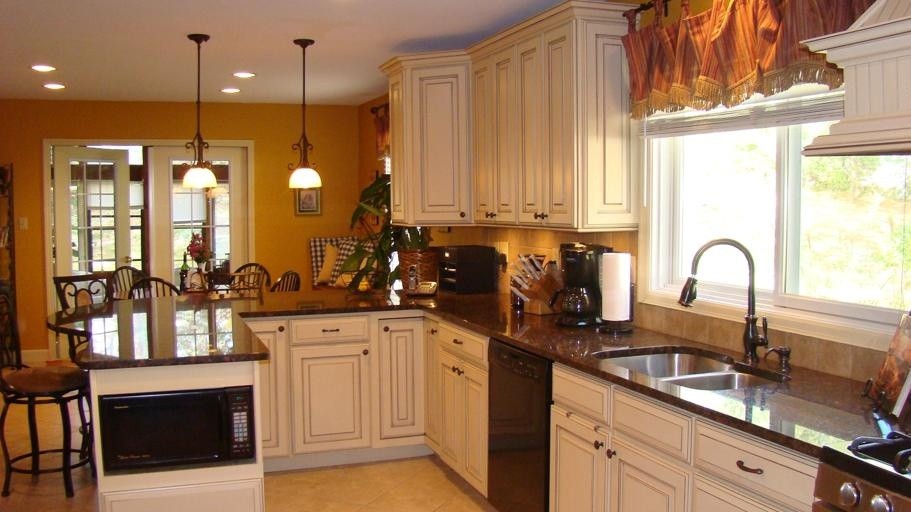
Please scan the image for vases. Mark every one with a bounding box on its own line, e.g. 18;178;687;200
196;261;206;271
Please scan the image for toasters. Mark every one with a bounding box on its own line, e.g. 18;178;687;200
439;244;498;294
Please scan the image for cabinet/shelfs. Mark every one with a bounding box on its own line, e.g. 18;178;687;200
551;362;690;512
516;15;642;236
371;310;426;447
245;320;291;460
472;44;517;229
291;313;371;456
690;417;817;512
379;61;473;228
427;314;488;507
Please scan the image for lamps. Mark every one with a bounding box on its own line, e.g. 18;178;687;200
287;36;322;188
181;32;217;189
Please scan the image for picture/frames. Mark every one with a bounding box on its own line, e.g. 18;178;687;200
295;188;321;216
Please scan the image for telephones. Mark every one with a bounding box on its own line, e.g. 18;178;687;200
405;264;438;297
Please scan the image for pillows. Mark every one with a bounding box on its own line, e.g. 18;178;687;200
327;237;380;285
332;254;377;292
309;236;357;287
316;241;341;283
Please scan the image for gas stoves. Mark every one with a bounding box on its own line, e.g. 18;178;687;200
812;430;911;511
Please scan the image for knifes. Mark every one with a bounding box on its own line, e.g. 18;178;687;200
510;251;548;301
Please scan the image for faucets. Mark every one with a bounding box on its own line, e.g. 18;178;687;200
677;238;769;363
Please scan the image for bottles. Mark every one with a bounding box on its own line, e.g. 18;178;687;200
511;308;524;337
511;274;525;306
182;251;191;288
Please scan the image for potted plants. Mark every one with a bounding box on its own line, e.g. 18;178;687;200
349;166;446;298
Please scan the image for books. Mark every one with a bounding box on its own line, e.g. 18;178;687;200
868;313;911;418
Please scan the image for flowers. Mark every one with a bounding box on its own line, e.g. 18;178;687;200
186;232;216;264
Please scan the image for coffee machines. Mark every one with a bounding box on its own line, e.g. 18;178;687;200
555;240;614;328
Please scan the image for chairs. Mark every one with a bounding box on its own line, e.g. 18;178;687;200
127;277;181;299
269;270;300;292
0;292;96;498
113;265;145;300
228;263;270;291
51;271;113;460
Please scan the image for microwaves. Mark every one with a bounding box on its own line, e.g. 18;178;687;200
97;387;258;476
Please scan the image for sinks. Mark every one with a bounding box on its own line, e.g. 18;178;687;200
602;352;790;391
